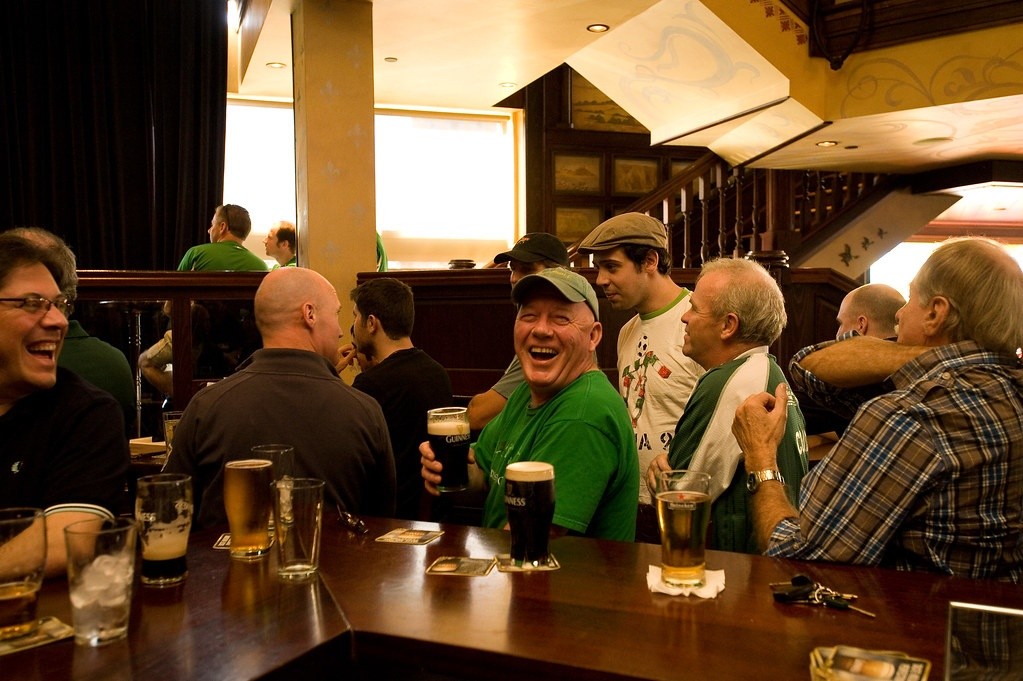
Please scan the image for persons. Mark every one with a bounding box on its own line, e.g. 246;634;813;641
334;276;455;522
577;213;708;545
646;258;809;553
419;266;640;544
0;230;132;584
732;236;1023;584
138;204;387;399
160;266;397;519
466;232;598;430
829;283;908;440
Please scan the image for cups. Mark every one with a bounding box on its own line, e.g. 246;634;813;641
427;407;471;493
223;460;273;562
63;517;139;647
250;443;294;530
505;461;556;569
135;474;193;589
270;478;325;580
0;508;48;643
163;411;183;460
655;470;712;590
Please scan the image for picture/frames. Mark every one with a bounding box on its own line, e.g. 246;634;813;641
550;149;607;198
567;66;650;135
549;197;607;248
666;149;727;207
610;151;664;197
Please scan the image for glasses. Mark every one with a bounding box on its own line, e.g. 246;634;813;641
0;294;75;317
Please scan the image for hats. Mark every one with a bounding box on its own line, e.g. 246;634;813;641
511;268;600;322
578;212;668;254
494;232;571;266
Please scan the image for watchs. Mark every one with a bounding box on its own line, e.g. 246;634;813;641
746;469;785;495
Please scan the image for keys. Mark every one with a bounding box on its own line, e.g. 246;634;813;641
768;573;875;618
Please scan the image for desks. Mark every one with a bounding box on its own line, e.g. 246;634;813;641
99;298;169;437
